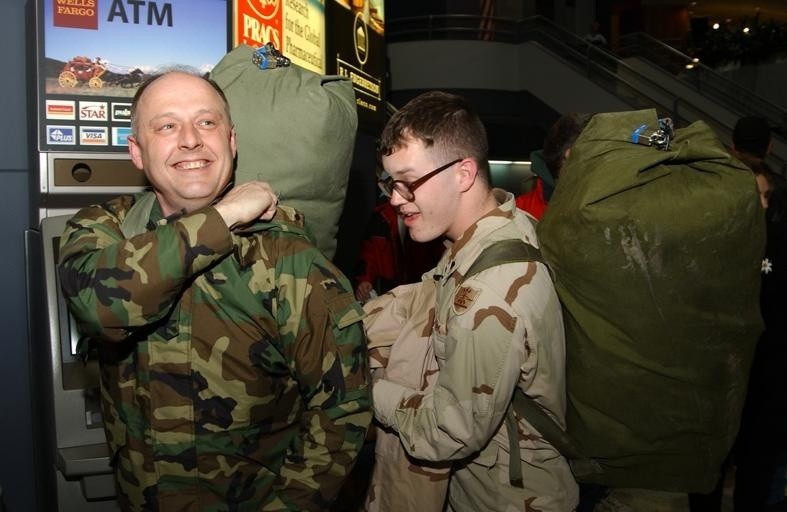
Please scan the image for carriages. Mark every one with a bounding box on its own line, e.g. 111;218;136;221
56;56;145;94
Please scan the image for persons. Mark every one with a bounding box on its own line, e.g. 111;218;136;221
53;70;373;510
510;109;595;222
583;23;607;45
360;88;582;511
350;192;446;305
683;114;787;512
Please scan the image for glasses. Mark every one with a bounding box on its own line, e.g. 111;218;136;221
377;159;464;202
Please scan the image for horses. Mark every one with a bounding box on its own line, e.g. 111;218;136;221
116;69;144;88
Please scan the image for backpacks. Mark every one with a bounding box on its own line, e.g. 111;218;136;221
461;106;767;495
74;42;359;430
